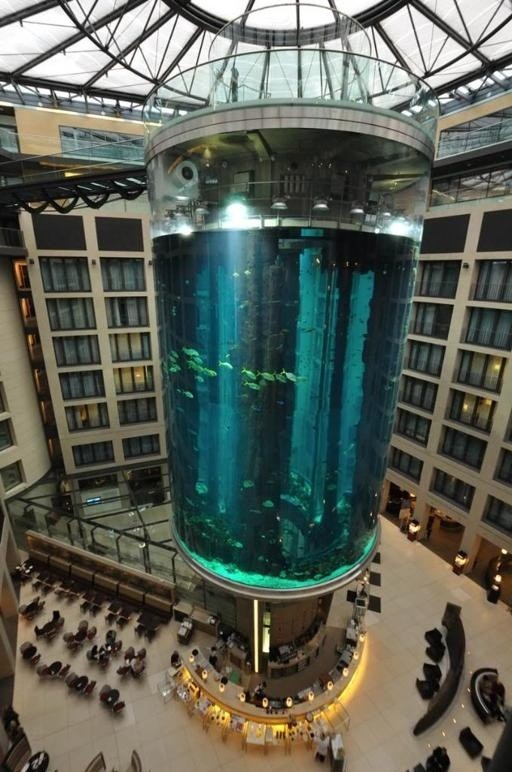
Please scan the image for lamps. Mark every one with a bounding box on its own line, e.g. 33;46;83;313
261;634;366;707
160;194;411;226
217;683;226;693
200;670;209;683
451;549;469;577
407;518;420;542
239;692;247;705
188;653;194;665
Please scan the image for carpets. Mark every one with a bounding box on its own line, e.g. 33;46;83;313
372;551;381;563
370;570;381;586
345;590;382;612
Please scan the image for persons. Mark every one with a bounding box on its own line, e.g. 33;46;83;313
254;681;267;698
313;731;331;762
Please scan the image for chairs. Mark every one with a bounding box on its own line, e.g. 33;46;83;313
422;744;450;772
17;566;162;715
415;623;446;701
458;724;485;760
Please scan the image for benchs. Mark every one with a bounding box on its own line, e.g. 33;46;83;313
471;664;499;723
442;599;463;623
4;736;32;770
125;749;142;771
85;752;106;772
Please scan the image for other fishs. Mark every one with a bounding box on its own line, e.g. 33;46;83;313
161;347;309;404
156;253;378;569
174;457;364;560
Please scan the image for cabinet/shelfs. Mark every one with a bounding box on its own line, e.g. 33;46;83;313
190;606;221;636
175;618;194;644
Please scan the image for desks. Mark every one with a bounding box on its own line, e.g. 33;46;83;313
21;750;51;771
